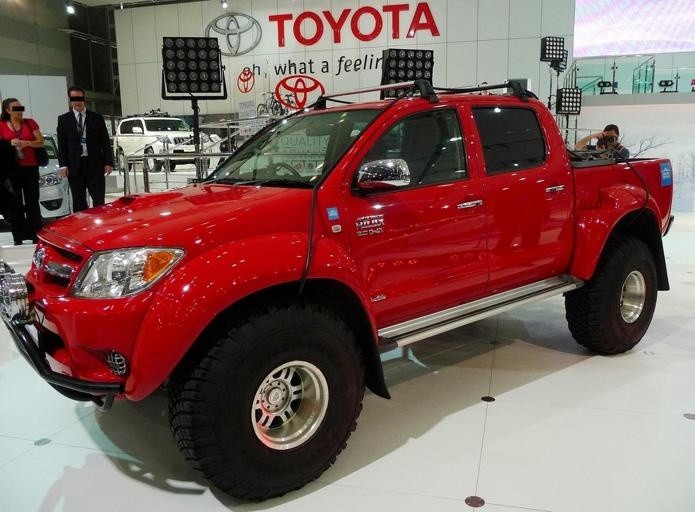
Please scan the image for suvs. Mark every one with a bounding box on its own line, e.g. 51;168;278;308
113;111;213;175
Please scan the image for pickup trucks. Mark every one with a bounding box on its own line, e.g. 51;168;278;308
0;75;679;506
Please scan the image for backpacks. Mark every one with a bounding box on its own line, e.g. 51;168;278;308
32;143;50;167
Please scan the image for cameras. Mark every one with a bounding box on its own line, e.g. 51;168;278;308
597;137;608;146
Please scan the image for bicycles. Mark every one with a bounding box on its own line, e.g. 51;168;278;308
274;94;299;116
256;92;284;119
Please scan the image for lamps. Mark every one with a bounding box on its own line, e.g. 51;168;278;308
379;48;435;102
540;37;582;149
160;36;228;182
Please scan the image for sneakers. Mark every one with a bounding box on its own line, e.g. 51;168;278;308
31;234;39;244
13;236;23;246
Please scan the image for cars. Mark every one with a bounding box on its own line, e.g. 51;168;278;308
1;131;91;232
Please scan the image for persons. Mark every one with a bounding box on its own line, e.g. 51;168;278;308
55;85;116;213
0;96;46;246
573;122;631;161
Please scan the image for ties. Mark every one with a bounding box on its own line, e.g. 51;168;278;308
78;112;83;148
